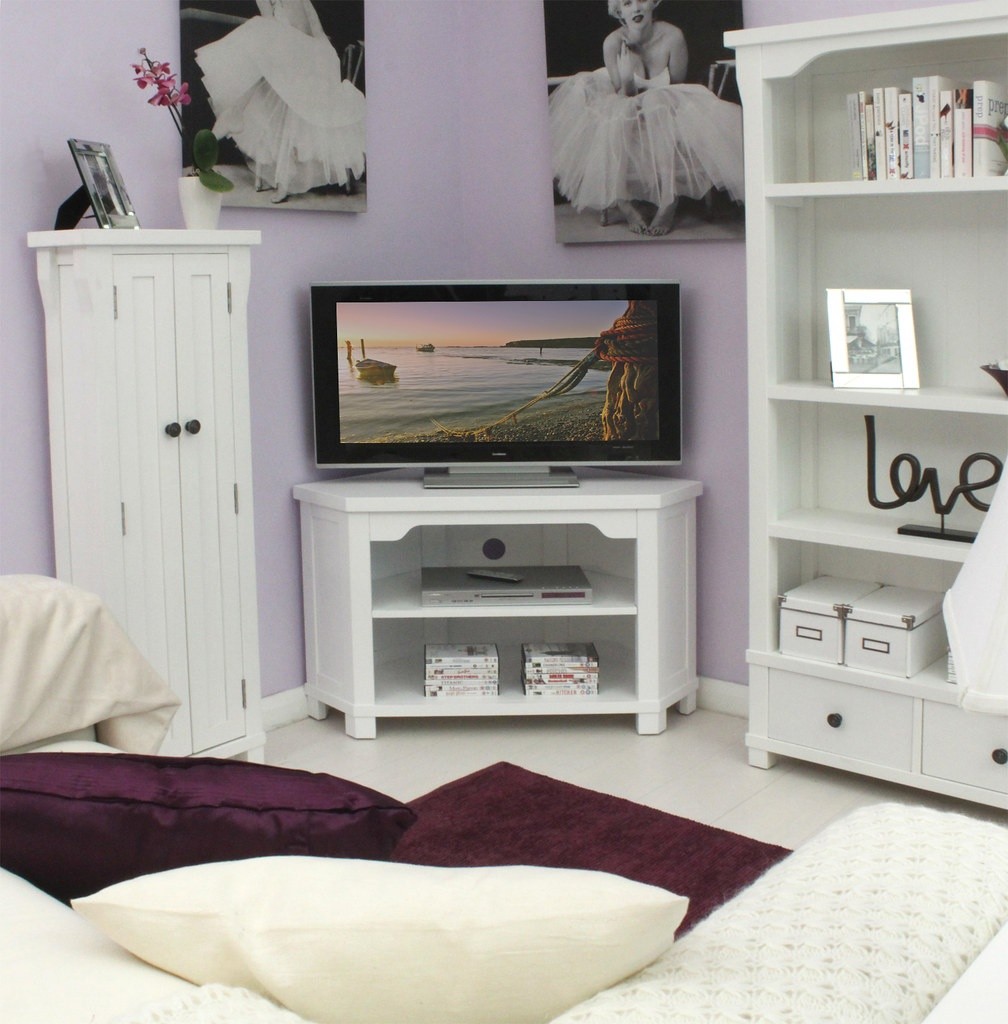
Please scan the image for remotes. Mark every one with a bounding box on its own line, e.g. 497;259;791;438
467;570;524;584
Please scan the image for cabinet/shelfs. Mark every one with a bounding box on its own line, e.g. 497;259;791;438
723;0;1008;814
29;230;263;763
293;466;704;739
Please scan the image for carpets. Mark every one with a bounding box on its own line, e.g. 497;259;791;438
391;761;793;942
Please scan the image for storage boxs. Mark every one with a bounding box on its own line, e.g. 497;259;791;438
843;585;950;678
778;576;884;664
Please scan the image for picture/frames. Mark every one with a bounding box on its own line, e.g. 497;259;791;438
67;139;141;229
826;289;920;389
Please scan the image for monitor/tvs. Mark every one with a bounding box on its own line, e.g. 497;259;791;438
311;278;682;488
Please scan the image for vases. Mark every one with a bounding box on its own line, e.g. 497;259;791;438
179;177;222;229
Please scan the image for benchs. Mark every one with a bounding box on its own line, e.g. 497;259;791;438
363;361;377;364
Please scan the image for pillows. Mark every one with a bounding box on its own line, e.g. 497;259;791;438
70;855;690;1024
1;752;418;907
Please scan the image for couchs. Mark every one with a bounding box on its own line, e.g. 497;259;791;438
1;572;1008;1024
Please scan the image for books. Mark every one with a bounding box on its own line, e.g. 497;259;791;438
846;75;1008;181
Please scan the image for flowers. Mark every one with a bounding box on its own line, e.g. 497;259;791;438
131;48;236;192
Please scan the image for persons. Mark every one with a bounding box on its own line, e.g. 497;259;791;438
546;0;745;237
193;0;367;203
